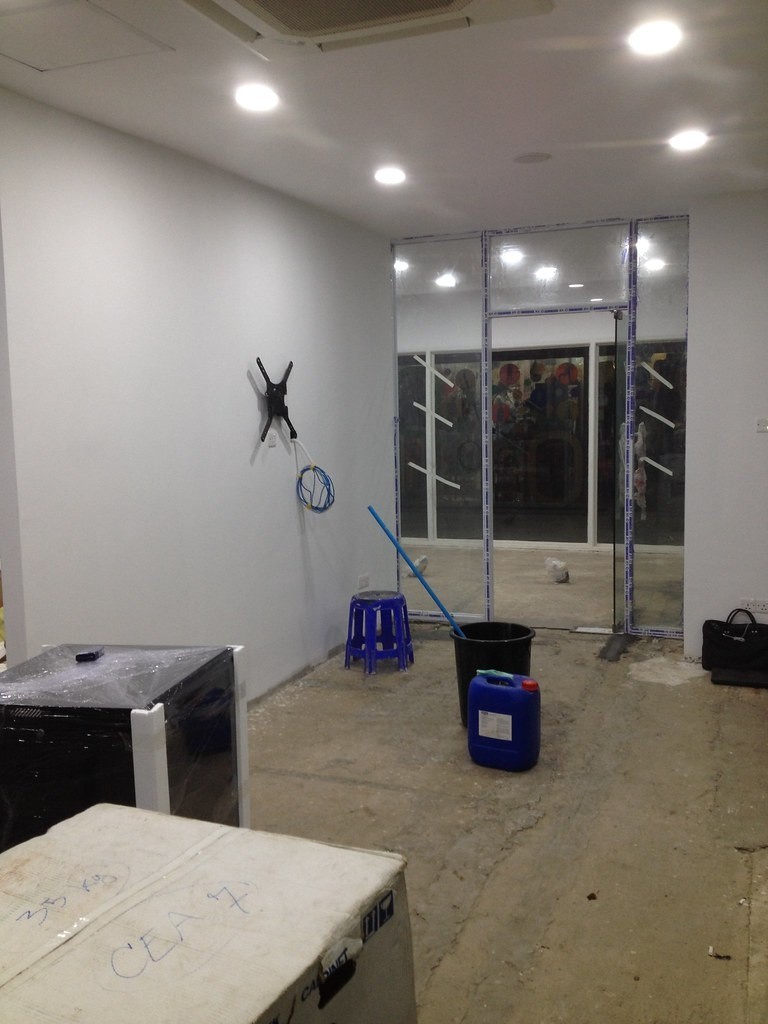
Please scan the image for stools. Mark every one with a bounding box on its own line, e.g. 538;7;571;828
344;590;415;675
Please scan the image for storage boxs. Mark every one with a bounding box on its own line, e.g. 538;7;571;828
0;802;418;1024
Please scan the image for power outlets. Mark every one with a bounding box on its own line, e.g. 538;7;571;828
741;599;756;613
756;600;768;613
268;432;277;448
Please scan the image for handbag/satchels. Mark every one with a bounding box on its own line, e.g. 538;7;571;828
702;608;768;689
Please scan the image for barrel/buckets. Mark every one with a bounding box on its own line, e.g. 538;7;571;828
449;620;535;728
465;669;541;771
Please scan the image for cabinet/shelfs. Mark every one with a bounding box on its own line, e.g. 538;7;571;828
0;642;250;829
